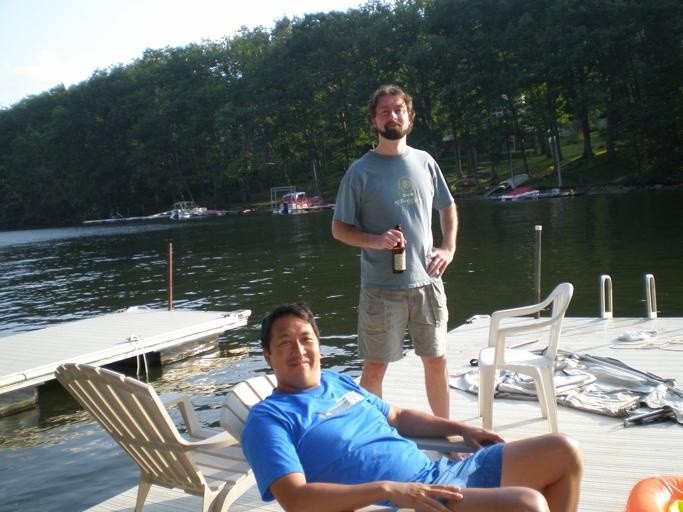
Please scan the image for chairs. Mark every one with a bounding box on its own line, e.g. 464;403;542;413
54;363;256;512
476;281;575;437
219;372;489;511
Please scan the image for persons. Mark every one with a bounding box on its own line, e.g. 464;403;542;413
331;85;467;463
238;299;583;511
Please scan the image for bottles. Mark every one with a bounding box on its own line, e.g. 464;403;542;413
392;224;407;274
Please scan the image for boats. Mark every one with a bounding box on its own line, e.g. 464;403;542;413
170;208;191;221
625;475;683;512
